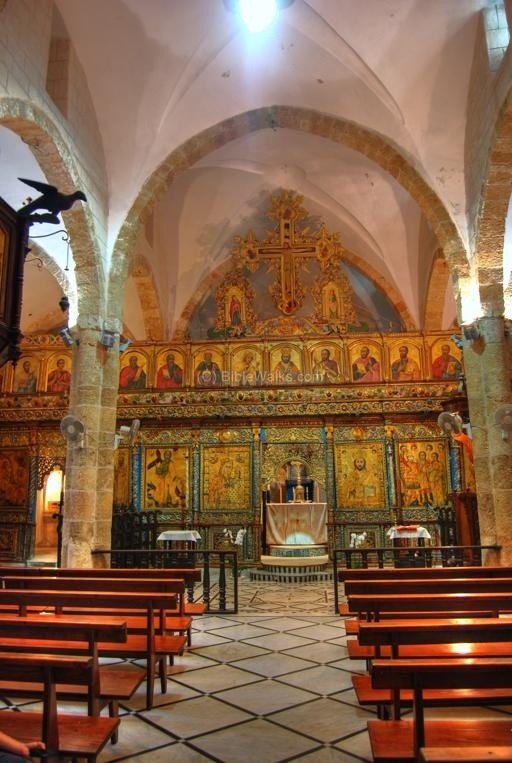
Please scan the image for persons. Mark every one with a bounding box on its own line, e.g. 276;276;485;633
12;361;37;393
118;343;465;389
341;456;382;508
147;450;181;506
47;359;71;393
399;441;446;505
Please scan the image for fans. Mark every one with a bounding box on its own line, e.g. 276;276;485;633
114;419;140;450
438;412;472;440
494;404;512;440
60;415;85;449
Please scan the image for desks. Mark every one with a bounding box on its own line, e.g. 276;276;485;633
157;530;201;567
386;526;431;567
265;502;329;545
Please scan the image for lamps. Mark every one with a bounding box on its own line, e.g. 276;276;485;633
100;333;119;347
451;334;463;350
460;320;479;340
120;338;133;351
58;328;74;346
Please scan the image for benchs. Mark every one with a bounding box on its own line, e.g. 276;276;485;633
336;568;512;763
0;566;208;763
